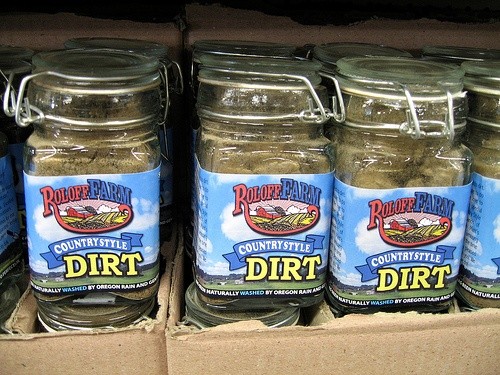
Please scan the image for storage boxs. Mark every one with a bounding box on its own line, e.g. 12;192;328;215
0;12;500;374
